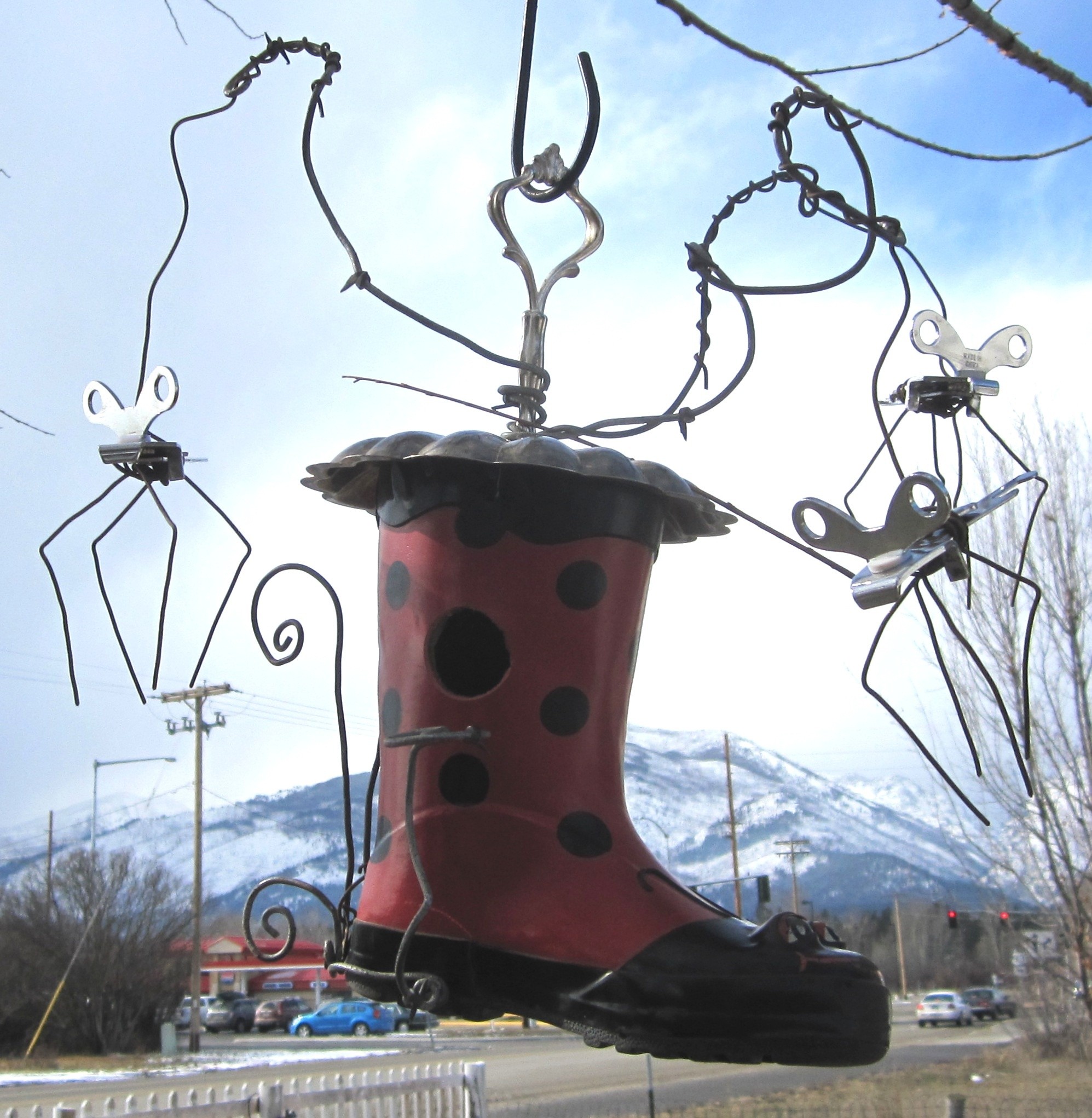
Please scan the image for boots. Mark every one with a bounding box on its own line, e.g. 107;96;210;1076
339;468;889;1065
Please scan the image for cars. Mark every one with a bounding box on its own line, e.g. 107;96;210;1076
253;995;314;1032
916;991;972;1027
204;989;267;1034
169;996;215;1029
289;1001;397;1039
383;1001;440;1035
962;986;1017;1022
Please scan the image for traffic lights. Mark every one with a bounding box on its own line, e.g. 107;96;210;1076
949;909;959;929
1000;910;1010;928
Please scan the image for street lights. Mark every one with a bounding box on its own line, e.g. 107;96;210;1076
801;900;815;922
89;757;176;869
